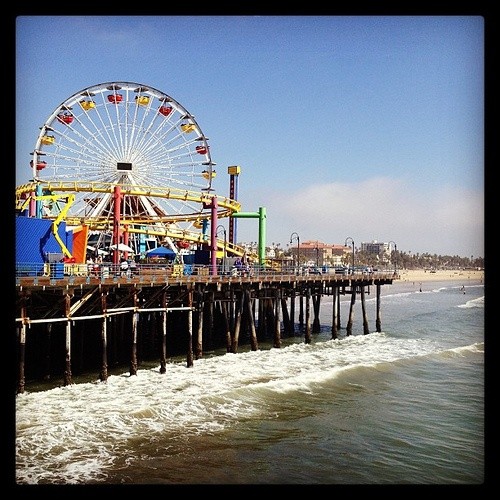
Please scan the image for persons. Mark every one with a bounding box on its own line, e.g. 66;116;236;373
120;251;137;278
366;265;374;276
235;252;254;277
87;255;103;264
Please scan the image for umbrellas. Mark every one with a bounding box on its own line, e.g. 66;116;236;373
146;244;175;270
109;243;134;265
